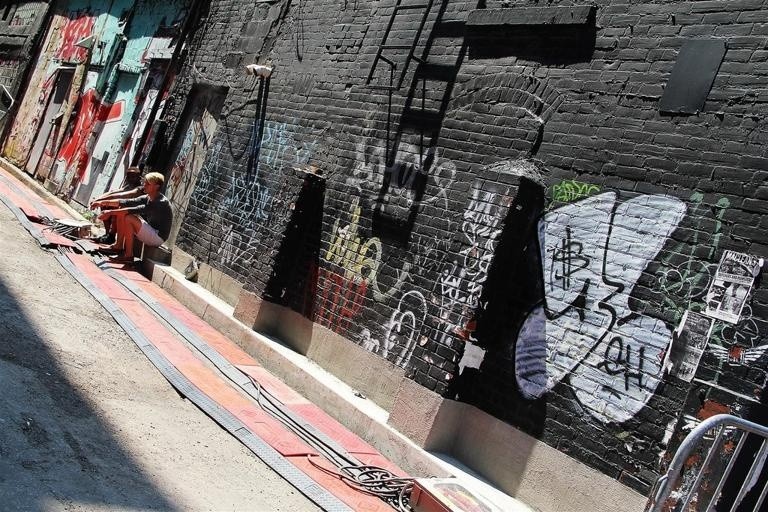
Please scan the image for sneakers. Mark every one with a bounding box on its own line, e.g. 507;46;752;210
90;235;134;265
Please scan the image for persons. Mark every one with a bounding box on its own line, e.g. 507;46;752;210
90;172;172;263
93;166;141;245
